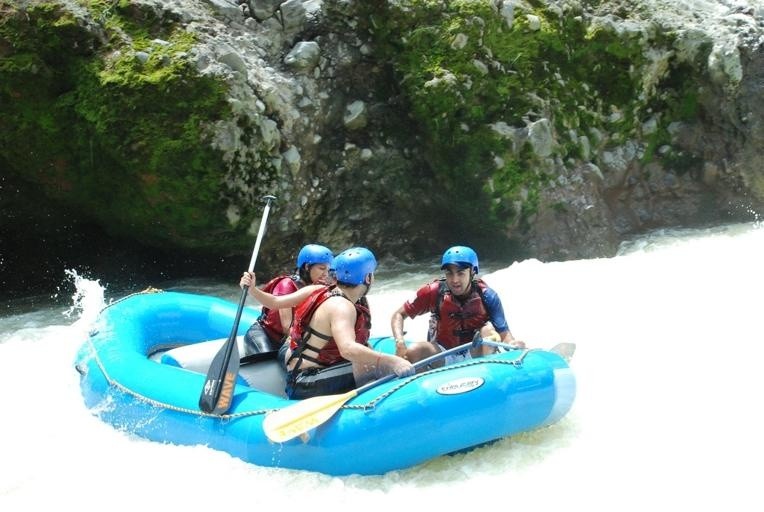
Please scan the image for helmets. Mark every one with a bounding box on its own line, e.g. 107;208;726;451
296;244;332;270
335;246;377;285
330;254;340;273
439;245;480;275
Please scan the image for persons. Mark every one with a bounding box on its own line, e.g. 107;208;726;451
238;269;331;371
242;244;334;362
286;248;413;403
391;244;525;373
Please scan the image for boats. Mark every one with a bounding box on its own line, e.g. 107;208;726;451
72;285;581;482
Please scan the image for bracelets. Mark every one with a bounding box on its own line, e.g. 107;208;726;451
374;352;385;377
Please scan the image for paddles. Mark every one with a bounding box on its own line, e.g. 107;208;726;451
199;195;277;413
472;331;574;366
262;335;492;442
